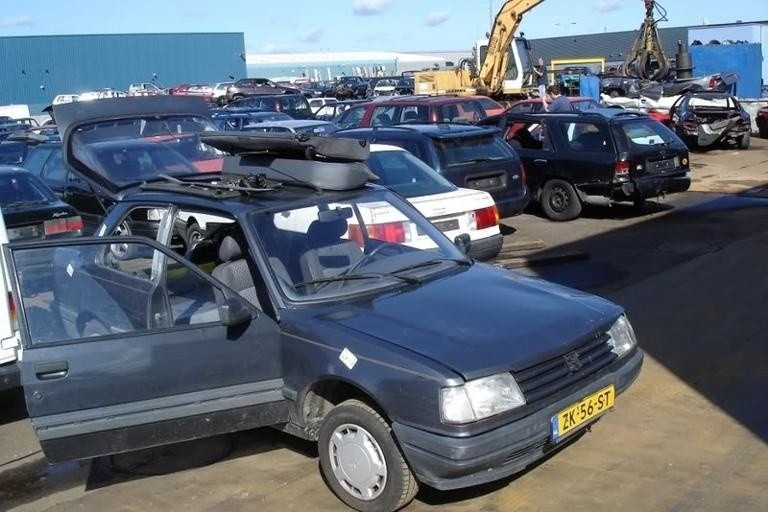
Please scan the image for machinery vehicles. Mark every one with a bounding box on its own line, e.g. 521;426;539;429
416;0;545;99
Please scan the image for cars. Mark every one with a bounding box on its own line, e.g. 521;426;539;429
171;78;301;101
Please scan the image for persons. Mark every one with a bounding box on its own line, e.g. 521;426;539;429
542;84;574;140
532;56;548;98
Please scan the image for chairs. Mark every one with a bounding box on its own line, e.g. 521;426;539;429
211;219;365;315
